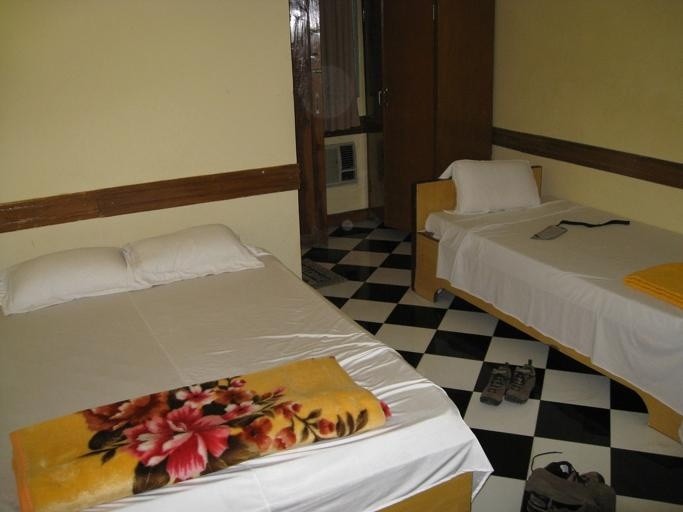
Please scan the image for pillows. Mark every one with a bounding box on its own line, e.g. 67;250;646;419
0;247;151;316
122;223;265;287
439;158;541;216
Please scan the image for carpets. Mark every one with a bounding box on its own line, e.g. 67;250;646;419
302;260;349;290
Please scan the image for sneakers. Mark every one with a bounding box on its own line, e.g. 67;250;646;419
479;359;537;406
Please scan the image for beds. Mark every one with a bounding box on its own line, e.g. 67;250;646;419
0;245;472;512
411;166;683;447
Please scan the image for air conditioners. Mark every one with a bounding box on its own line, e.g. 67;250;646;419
324;133;370;215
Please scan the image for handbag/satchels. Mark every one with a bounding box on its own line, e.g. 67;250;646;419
519;450;616;512
